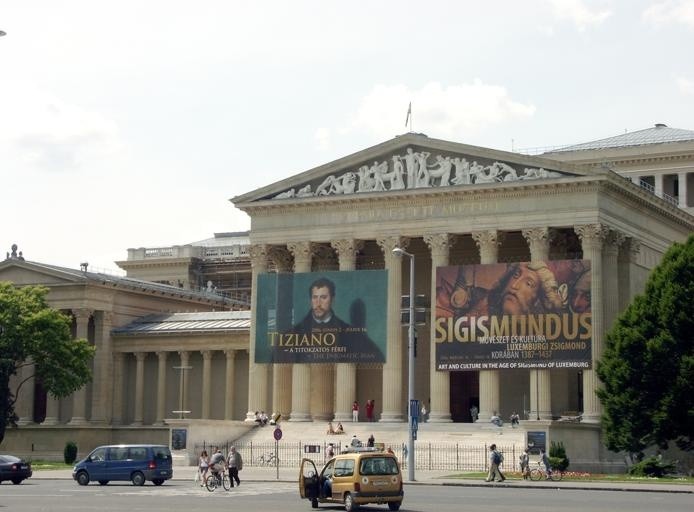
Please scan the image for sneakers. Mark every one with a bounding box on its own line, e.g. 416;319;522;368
230;481;240;487
485;478;506;482
200;482;206;487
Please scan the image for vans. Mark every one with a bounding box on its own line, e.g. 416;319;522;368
299;447;404;512
72;444;173;486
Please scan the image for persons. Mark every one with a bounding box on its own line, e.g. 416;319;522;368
275;278;385;363
570;269;591;314
469;404;478;422
207;449;227;487
519;448;529;476
490;443;506;481
196;449;211;487
364;399;373;422
321;419;394;464
224;446;243;487
490;411;502;426
269;146;563;200
320;474;331;498
350;400;359;422
253;411;264;427
259;411;268;424
484;445;502;482
510;410;519;424
441;262;565;350
537;448;552;479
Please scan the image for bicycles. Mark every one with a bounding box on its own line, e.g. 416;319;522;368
256;453;279;467
205;463;231;492
529;462;562;482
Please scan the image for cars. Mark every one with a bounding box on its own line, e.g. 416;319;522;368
0;455;32;484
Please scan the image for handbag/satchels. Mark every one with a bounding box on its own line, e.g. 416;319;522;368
522;471;530;476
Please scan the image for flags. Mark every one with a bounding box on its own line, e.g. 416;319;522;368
404;102;411;127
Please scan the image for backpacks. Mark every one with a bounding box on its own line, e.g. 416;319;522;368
492;450;500;465
497;452;504;462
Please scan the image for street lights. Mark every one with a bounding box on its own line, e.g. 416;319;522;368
392;247;415;480
